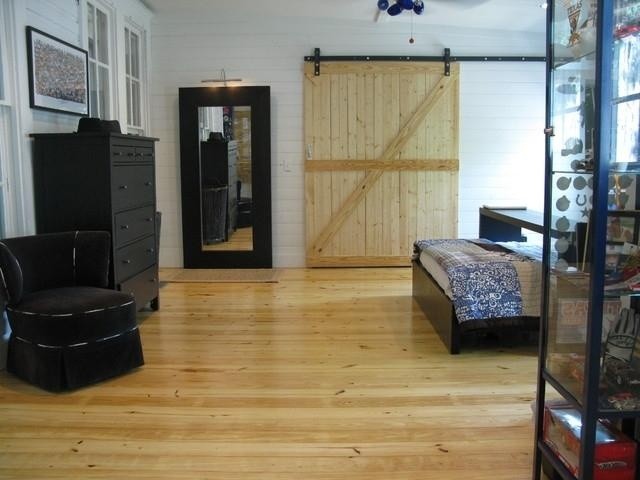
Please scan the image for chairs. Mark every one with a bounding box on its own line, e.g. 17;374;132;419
238;181;253;228
0;230;145;393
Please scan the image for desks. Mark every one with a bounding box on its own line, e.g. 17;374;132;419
478;205;582;267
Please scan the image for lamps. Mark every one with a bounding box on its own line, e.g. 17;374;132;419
200;68;242;87
372;0;425;44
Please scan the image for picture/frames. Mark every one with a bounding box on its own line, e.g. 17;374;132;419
26;25;90;116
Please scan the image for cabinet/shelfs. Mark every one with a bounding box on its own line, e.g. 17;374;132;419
29;131;160;310
201;140;238;242
533;0;640;480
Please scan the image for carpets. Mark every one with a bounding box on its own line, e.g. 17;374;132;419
160;269;279;283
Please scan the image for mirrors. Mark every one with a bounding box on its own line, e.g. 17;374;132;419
179;86;271;269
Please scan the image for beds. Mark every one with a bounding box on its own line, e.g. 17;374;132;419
411;239;559;354
